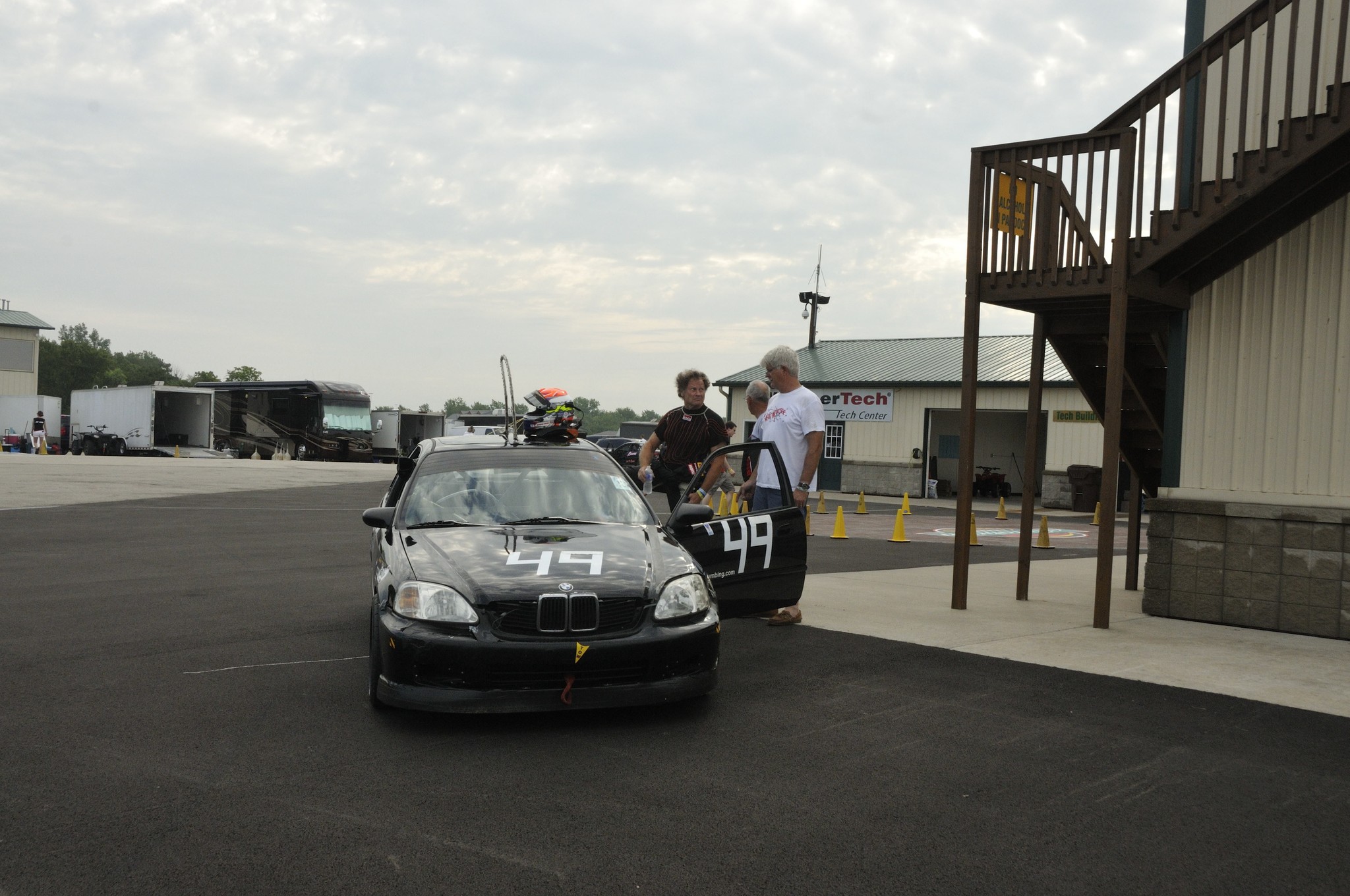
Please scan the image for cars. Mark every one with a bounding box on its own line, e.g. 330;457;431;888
586;435;661;467
61;414;70;455
362;434;806;713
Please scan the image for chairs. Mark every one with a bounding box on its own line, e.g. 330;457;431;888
425;478;601;517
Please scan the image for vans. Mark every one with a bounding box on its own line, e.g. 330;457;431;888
451;427;515;436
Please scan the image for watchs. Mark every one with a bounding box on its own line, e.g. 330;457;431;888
798;482;810;489
729;469;733;472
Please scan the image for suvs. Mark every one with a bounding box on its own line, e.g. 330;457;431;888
71;425;127;456
972;466;1011;497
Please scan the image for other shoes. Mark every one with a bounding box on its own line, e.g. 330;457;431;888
767;610;802;625
755;609;779;618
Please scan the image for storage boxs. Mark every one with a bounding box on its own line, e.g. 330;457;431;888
3;434;22;444
168;434;189;445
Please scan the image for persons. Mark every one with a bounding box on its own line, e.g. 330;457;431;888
638;434;646;443
701;421;737;516
638;370;730;528
736;344;825;625
30;411;48;455
741;379;771;518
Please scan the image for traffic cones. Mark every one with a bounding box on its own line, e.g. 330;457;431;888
708;491;730;517
995;497;1008;519
813;491;829;514
901;492;912;515
806;505;849;538
854;492;869;514
1089;502;1099;525
174;445;180;457
39;440;48;454
887;509;911;542
729;493;748;516
1032;516;1055;549
970;513;983;546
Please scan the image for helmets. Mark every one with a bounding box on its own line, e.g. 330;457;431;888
523;388;576;440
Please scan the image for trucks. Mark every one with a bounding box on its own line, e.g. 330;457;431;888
193;380;383;462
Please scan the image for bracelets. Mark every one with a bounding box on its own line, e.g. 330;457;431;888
797;485;808;492
31;431;33;433
697;488;706;499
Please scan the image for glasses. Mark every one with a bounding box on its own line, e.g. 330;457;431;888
743;395;750;401
764;366;779;377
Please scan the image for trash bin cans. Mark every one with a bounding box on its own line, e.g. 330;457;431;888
1067;464;1102;513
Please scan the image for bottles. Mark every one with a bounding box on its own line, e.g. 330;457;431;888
643;466;653;494
